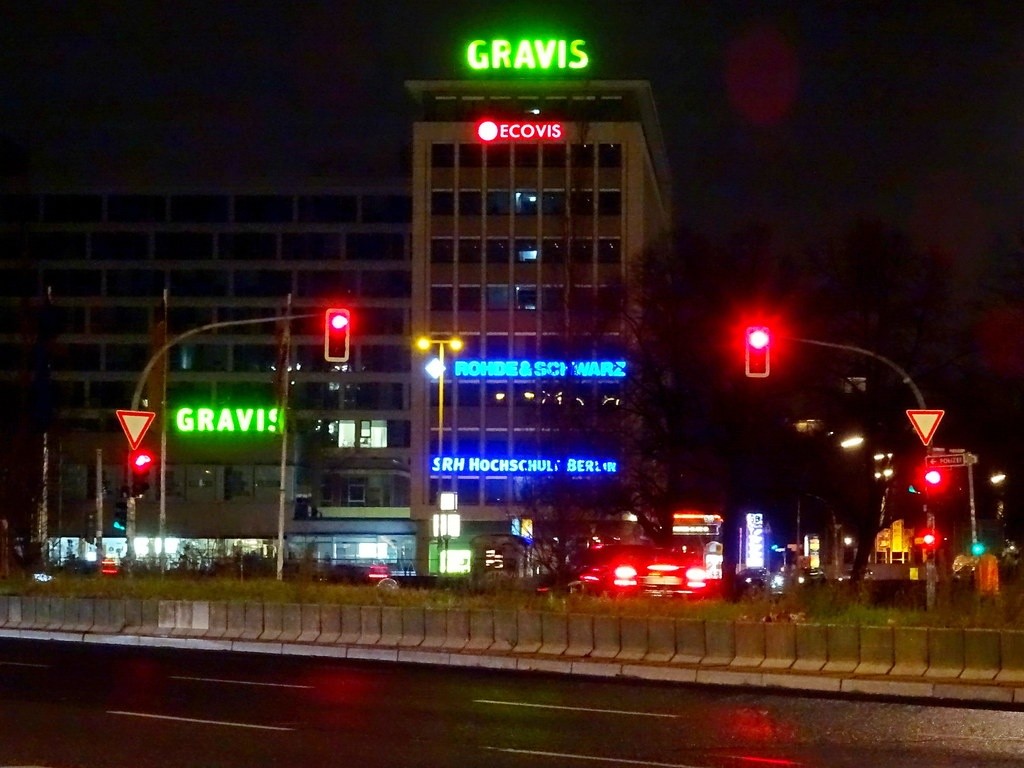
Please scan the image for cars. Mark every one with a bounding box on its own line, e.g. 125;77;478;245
331;562;394;587
60;557;120;577
535;538;712;599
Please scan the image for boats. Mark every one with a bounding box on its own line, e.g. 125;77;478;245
131;451;156;472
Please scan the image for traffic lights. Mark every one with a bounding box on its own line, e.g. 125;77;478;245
972;542;984;561
922;532;940;550
746;328;770;376
925;467;948;487
324;309;353;362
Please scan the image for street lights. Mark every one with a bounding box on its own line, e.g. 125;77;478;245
415;338;463;578
